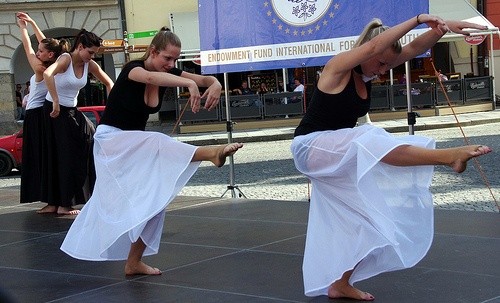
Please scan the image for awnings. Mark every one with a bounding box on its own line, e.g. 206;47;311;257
168;0;500;111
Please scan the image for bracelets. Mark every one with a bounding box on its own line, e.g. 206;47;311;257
416;14;421;25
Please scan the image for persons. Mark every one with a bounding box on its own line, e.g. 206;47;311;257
15;81;30;119
256;82;271;94
402;74;406;84
41;29;114;215
275;79;293;93
16;11;66;214
434;69;448;81
290;13;492;300
59;26;244;278
232;80;255;95
293;77;305;97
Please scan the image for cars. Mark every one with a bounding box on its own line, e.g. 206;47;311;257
0;105;106;177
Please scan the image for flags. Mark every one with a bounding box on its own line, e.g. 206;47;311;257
197;0;432;75
